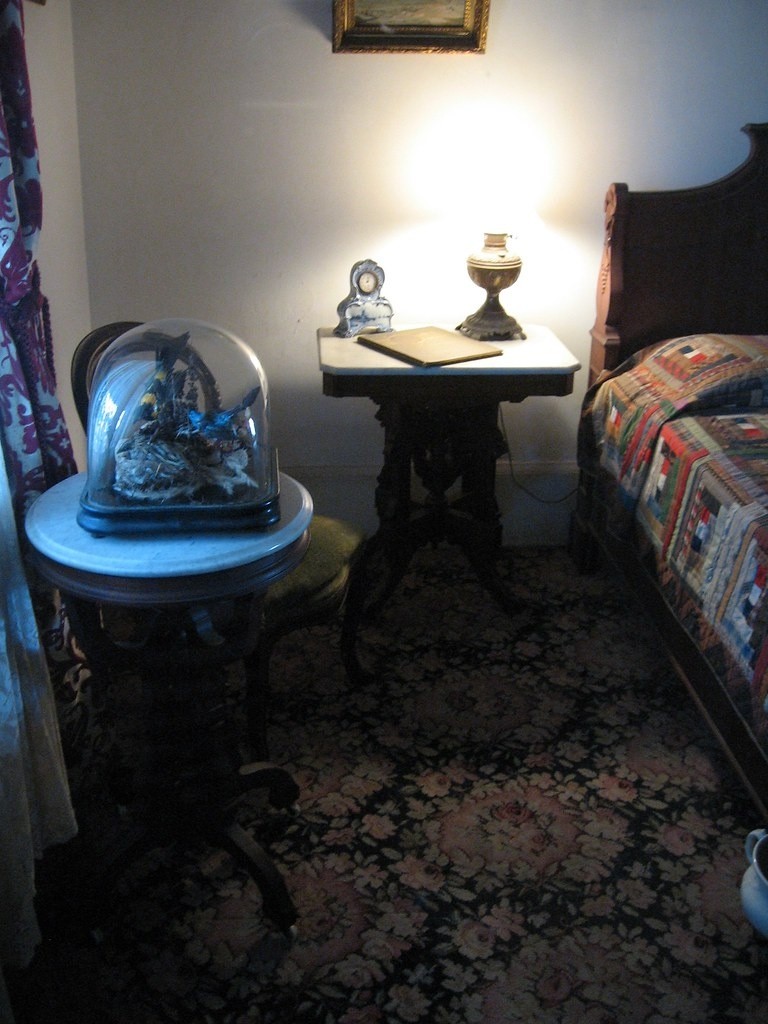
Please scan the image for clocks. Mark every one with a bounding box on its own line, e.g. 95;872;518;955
333;258;395;339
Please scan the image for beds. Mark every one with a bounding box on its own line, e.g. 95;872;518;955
565;124;768;818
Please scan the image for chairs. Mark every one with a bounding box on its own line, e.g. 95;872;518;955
71;322;364;752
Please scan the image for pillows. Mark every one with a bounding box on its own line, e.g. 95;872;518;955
576;330;768;543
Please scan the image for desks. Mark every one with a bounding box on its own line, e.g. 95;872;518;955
314;314;585;581
23;468;315;929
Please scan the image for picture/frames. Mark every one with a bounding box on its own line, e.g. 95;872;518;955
330;0;490;54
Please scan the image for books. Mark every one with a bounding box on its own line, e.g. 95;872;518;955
358;327;503;367
